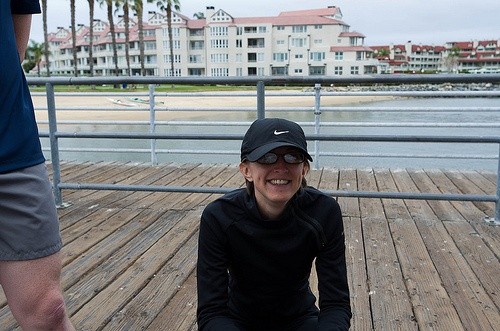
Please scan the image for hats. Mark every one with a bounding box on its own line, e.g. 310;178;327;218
240;118;313;163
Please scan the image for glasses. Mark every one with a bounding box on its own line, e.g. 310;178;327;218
246;152;305;164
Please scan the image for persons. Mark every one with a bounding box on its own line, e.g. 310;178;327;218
0;0;75;331
197;118;353;331
